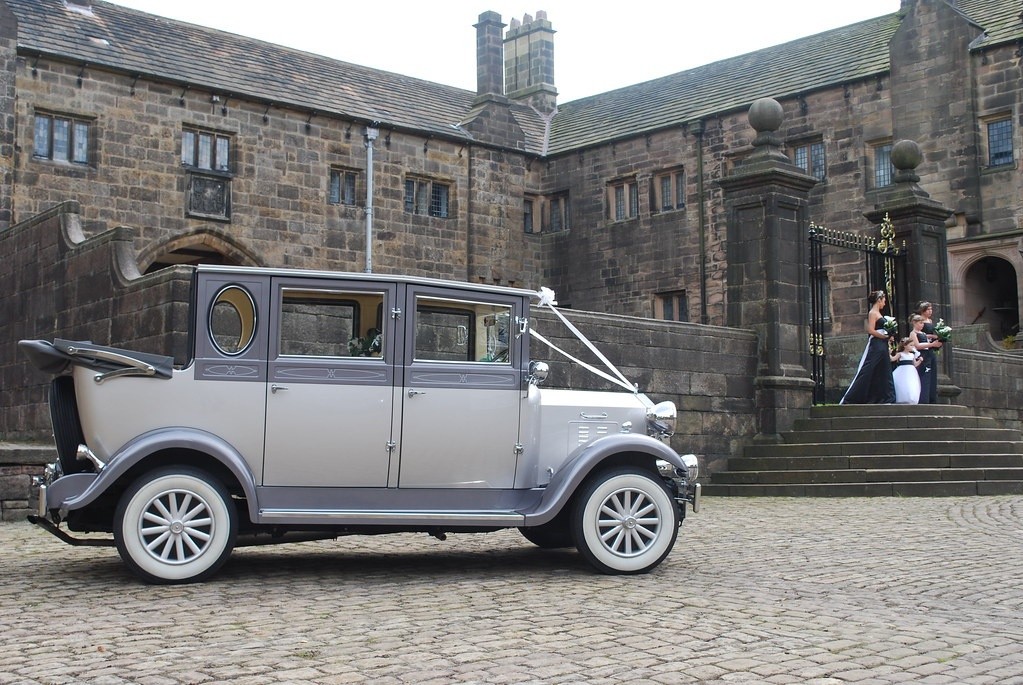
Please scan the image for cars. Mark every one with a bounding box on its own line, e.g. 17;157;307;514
18;262;702;585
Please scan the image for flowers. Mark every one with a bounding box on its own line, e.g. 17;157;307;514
934;318;953;355
884;316;898;333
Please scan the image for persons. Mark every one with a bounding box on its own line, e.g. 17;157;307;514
909;301;942;404
839;290;896;404
890;337;923;404
